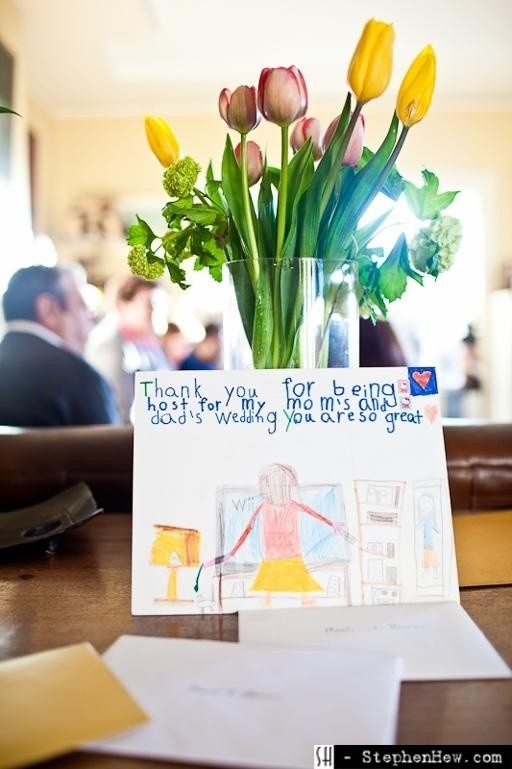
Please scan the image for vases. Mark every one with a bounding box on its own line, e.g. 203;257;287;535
222;256;359;368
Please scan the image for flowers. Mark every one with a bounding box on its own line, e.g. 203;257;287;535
125;18;465;368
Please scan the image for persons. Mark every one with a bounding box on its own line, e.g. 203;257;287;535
0;266;221;427
